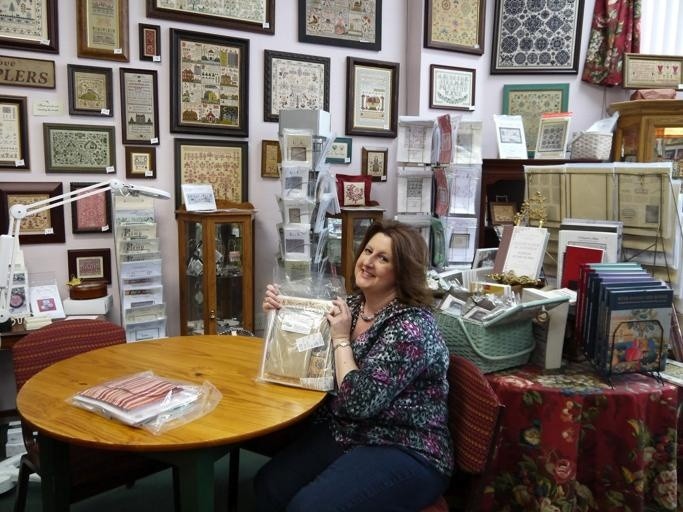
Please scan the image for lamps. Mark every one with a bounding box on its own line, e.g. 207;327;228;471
1;178;172;326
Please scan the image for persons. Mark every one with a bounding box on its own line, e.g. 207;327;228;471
254;220;455;512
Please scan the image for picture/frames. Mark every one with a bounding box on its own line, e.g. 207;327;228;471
1;0;683;285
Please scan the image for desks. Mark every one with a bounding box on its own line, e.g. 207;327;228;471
16;336;326;512
476;337;683;510
476;366;683;510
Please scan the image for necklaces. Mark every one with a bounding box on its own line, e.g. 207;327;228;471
360;297;400;321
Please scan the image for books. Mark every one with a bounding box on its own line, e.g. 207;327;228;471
522;287;569;370
492;225;550;281
113;193;167;343
557;219;675;377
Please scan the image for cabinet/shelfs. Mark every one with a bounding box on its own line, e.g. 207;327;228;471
174;200;257;335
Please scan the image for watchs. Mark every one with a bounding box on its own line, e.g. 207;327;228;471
333;341;351;350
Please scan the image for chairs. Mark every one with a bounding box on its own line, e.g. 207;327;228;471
422;356;505;512
11;320;182;512
